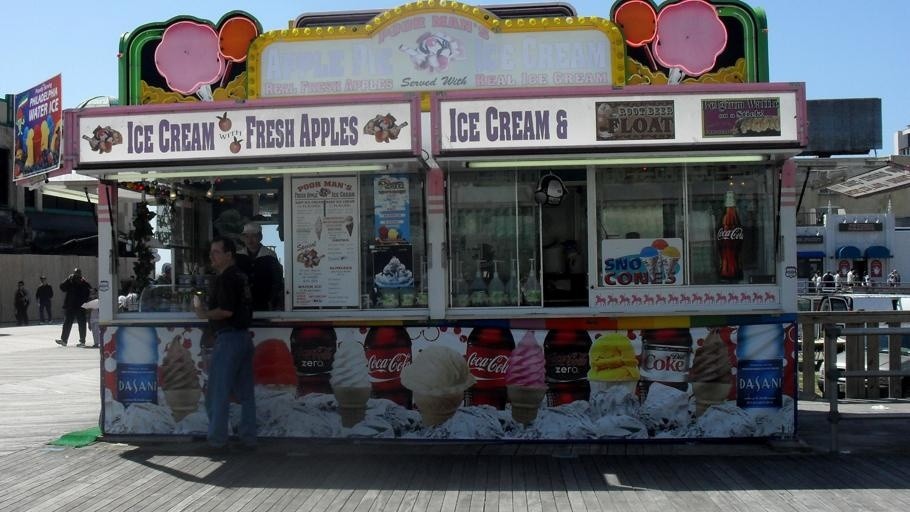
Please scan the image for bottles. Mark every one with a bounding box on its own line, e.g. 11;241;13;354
735;324;785;416
289;325;338;400
115;326;159;409
543;327;594;407
362;326;414;412
463;327;517;412
714;189;746;284
638;328;693;407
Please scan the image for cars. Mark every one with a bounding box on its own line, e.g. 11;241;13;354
797;293;910;399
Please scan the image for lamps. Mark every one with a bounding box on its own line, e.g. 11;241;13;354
169;183;176;200
206;181;216;198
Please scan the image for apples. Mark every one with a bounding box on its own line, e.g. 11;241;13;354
378;226;387;241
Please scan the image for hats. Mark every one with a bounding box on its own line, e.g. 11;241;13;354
242;222;262;235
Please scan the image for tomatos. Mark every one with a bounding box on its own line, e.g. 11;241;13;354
230;137;243;154
216;112;232;131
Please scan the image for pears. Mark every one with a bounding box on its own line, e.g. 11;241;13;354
388;228;399;240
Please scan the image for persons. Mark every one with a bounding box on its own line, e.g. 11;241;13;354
86;287;100;347
117;273;172;314
811;268;901;292
234;223;283;270
13;281;30;324
192;237;259;455
35;276;54;325
55;267;92;346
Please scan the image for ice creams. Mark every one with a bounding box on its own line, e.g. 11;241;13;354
639;239;680;282
585;332;641;402
328;340;373;428
690;329;735;417
376;256;413;287
160;335;203;423
400;346;475;427
505;329;549;426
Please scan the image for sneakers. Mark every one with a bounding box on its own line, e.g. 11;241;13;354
77;342;85;347
56;339;67;346
240;434;258;448
